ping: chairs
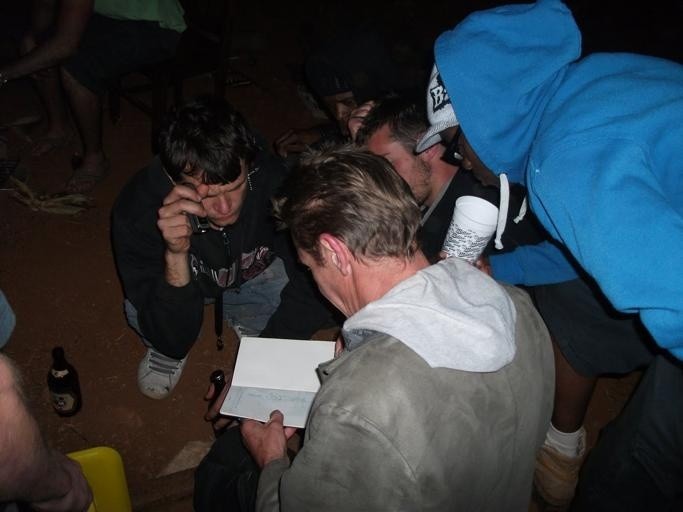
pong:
[106,14,230,157]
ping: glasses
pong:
[440,125,465,167]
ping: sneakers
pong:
[534,430,587,506]
[137,347,191,399]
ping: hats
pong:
[416,63,460,153]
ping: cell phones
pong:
[180,181,210,234]
